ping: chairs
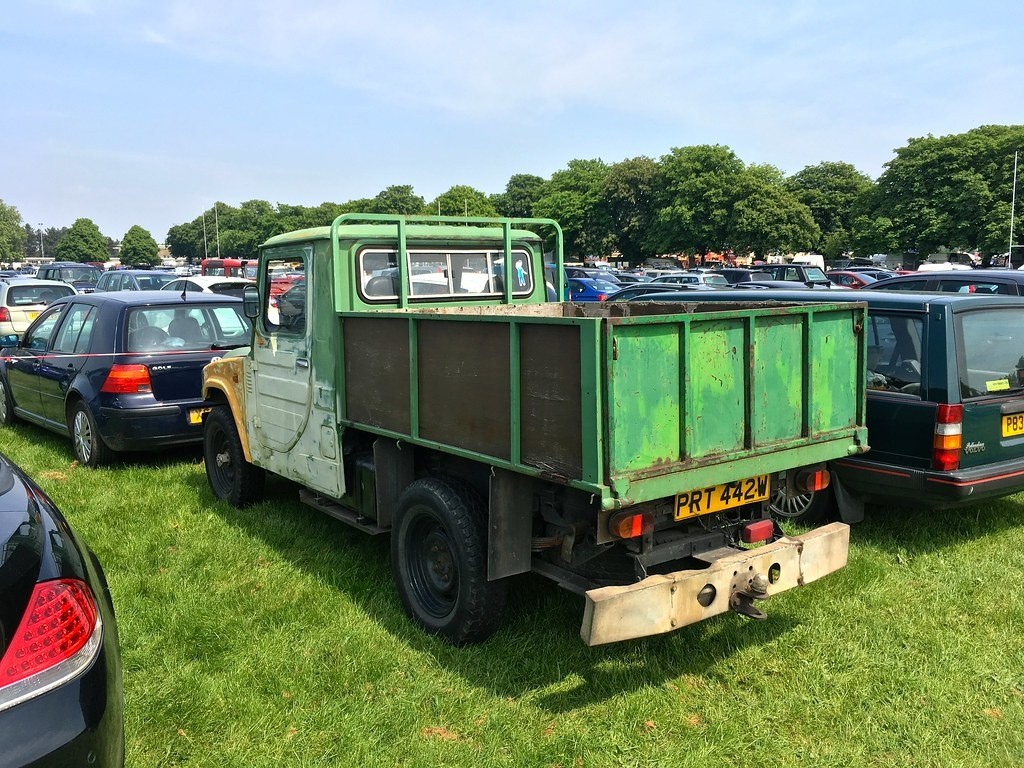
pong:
[366,275,401,298]
[484,274,515,292]
[168,315,204,342]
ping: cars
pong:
[1,260,1024,522]
[0,450,124,768]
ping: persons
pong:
[895,262,903,271]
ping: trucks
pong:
[194,212,872,646]
